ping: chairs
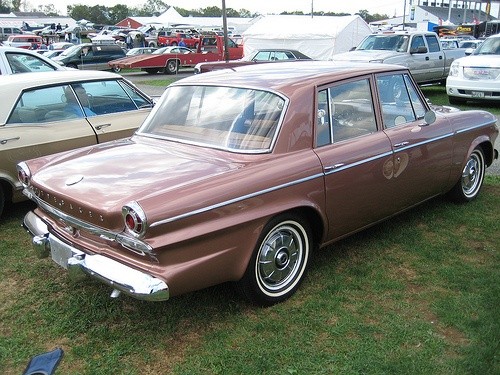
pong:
[18,105,78,123]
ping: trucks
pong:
[108,35,244,75]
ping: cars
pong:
[0,69,163,220]
[126,47,159,57]
[438,28,486,56]
[445,31,500,106]
[16,59,500,307]
[0,23,240,51]
[193,48,313,75]
[0,46,79,76]
[151,46,195,55]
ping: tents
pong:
[243,15,373,61]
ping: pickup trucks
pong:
[329,31,466,85]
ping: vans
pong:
[43,42,127,73]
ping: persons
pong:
[125,34,133,49]
[178,38,186,47]
[57,23,69,30]
[65,85,96,117]
[150,25,156,32]
